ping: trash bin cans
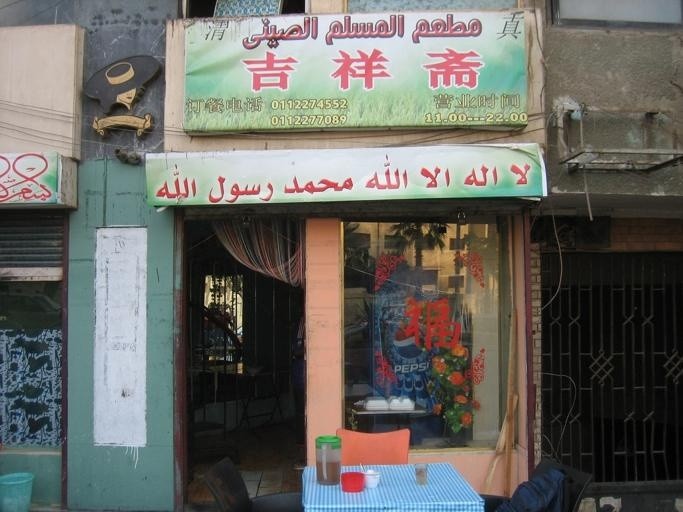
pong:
[0,472,35,512]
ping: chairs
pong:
[479,457,596,510]
[202,454,301,511]
[332,424,411,466]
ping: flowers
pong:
[398,296,481,438]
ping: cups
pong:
[414,463,428,484]
[315,435,380,491]
